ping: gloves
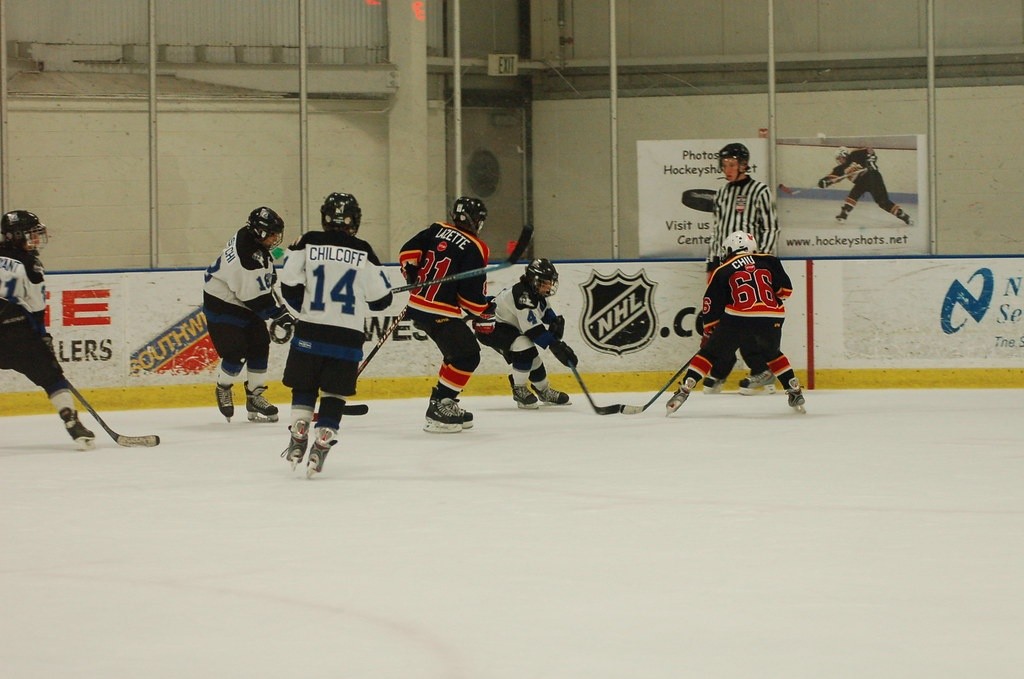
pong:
[273,308,295,329]
[700,332,710,349]
[819,178,832,188]
[868,161,878,172]
[549,341,578,368]
[473,303,497,336]
[548,315,564,340]
[41,333,52,346]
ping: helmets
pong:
[721,230,757,263]
[720,143,749,167]
[321,192,363,230]
[247,207,284,241]
[452,197,488,232]
[0,211,46,255]
[834,146,849,159]
[526,258,558,297]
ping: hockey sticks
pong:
[269,284,369,417]
[391,224,535,294]
[313,304,407,422]
[620,326,715,415]
[57,363,160,447]
[555,336,621,415]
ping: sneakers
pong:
[896,209,912,225]
[281,420,310,470]
[306,427,338,478]
[836,211,848,223]
[423,369,806,433]
[215,382,235,423]
[244,380,279,422]
[59,408,95,449]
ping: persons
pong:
[703,143,782,395]
[818,146,914,225]
[204,206,293,422]
[398,196,497,433]
[664,232,806,417]
[477,258,578,409]
[280,192,393,478]
[0,210,97,450]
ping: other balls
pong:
[660,327,670,337]
